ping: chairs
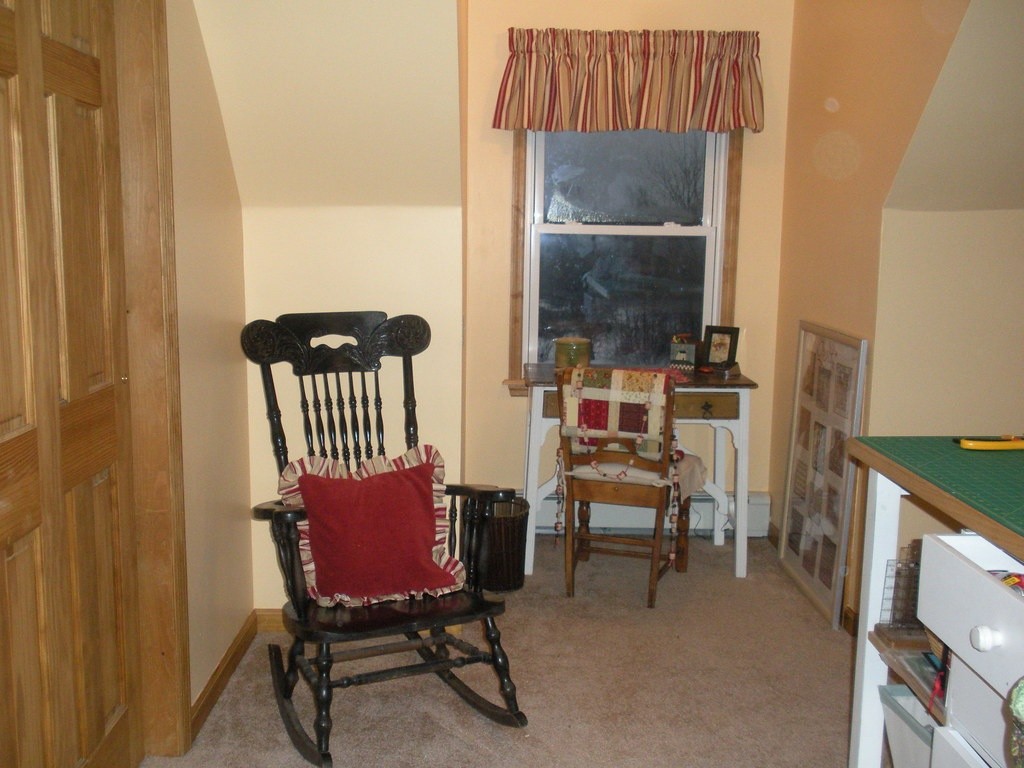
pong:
[553,364,704,609]
[239,309,528,768]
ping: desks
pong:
[843,431,1023,768]
[518,361,758,580]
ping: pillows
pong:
[276,442,466,608]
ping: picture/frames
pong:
[703,325,740,367]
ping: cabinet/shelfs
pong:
[916,526,1022,768]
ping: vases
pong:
[777,319,871,634]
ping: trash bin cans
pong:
[461,496,531,594]
